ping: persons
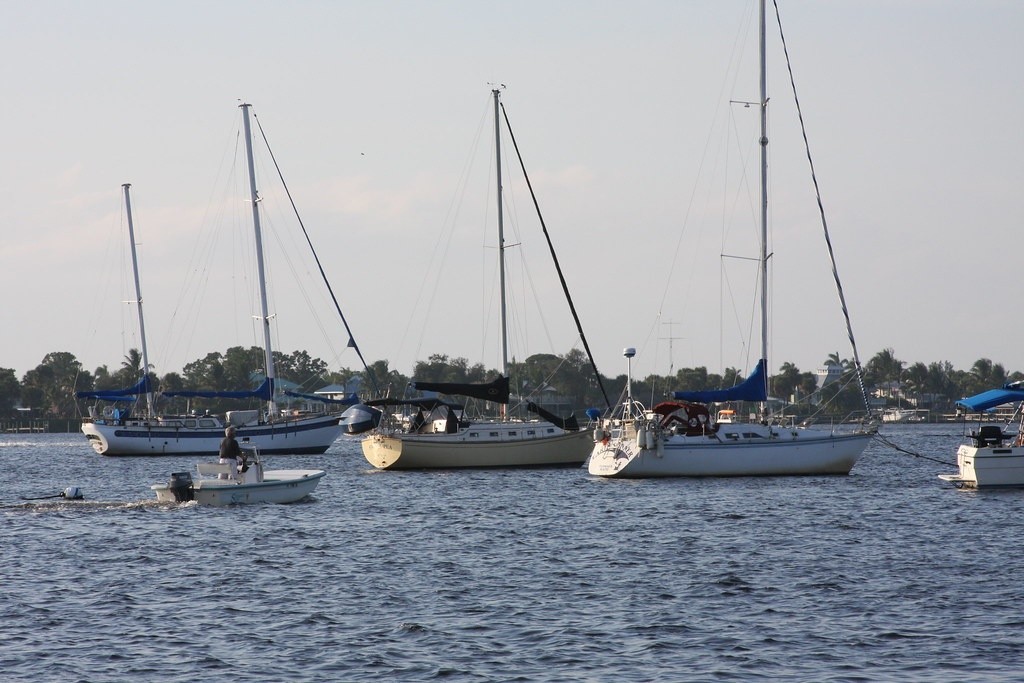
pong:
[191,409,213,418]
[219,426,249,473]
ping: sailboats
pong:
[586,0,885,478]
[80,89,395,458]
[362,79,625,471]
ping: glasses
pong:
[232,432,237,435]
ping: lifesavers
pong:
[103,405,114,418]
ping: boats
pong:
[935,378,1024,490]
[849,403,928,425]
[151,441,326,507]
